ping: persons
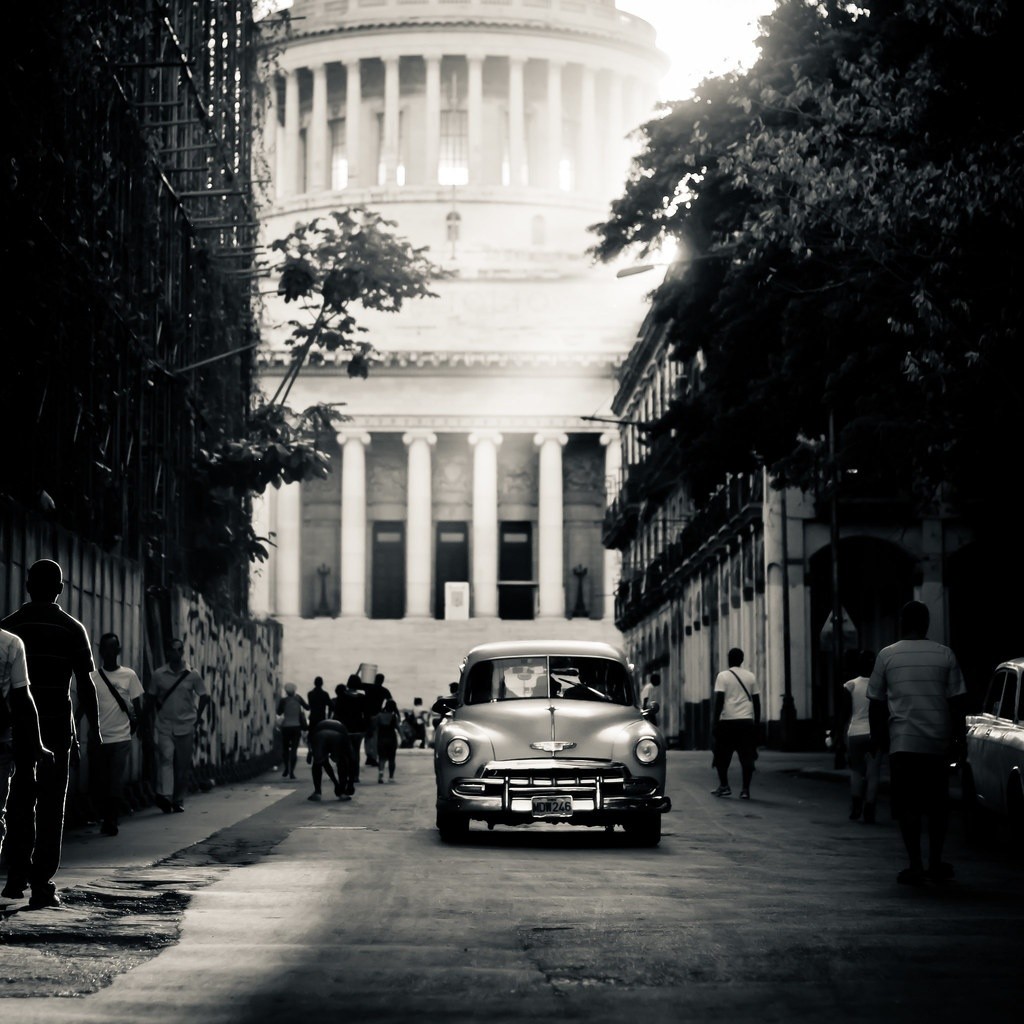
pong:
[866,600,969,888]
[0,559,208,906]
[831,649,881,823]
[710,648,760,798]
[640,674,661,726]
[276,673,458,802]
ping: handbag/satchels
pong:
[130,712,139,735]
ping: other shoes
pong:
[308,792,324,802]
[849,810,876,825]
[154,793,173,814]
[338,795,352,802]
[29,895,59,909]
[895,865,941,885]
[378,770,383,784]
[388,778,395,783]
[711,786,731,797]
[282,769,296,779]
[740,791,750,799]
[100,821,119,836]
[1,885,24,900]
[174,804,185,813]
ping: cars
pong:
[430,636,673,849]
[958,656,1024,849]
